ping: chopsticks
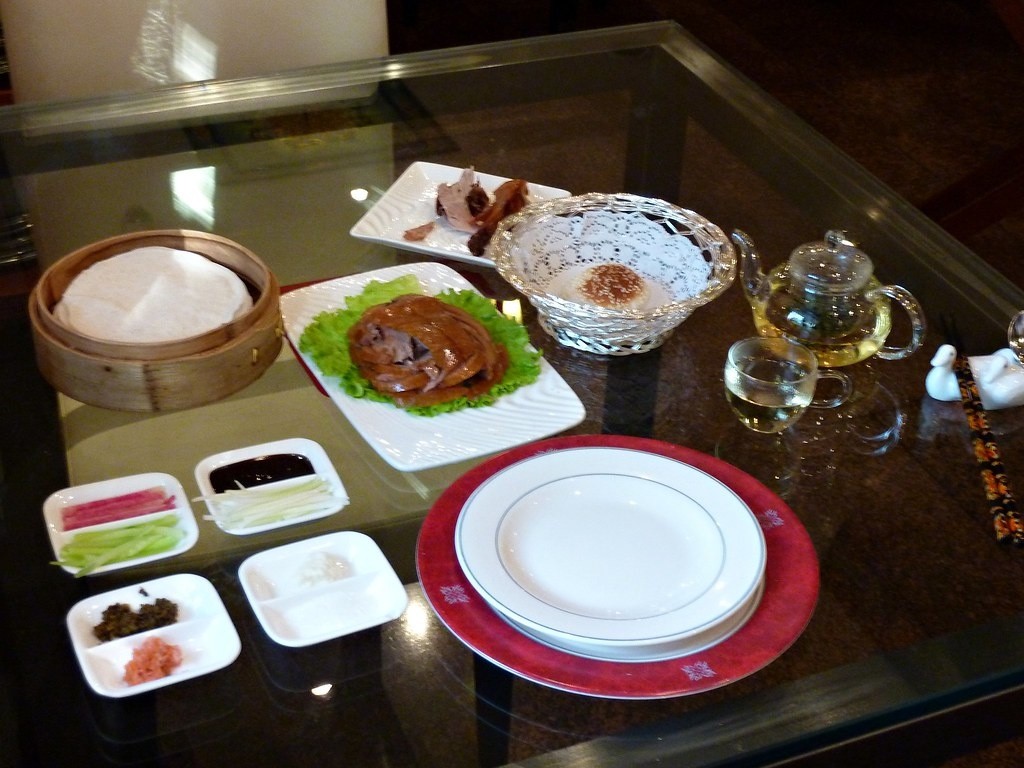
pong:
[938,307,1024,551]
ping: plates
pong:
[349,161,572,268]
[454,446,768,647]
[238,530,409,648]
[195,438,350,536]
[414,433,821,701]
[64,573,242,698]
[278,262,587,473]
[42,472,201,575]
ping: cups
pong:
[724,336,854,434]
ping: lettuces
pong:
[294,277,545,418]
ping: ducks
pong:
[402,165,532,257]
[347,293,510,408]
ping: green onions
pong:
[190,476,352,531]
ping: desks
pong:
[0,20,1024,768]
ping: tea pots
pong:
[732,227,927,370]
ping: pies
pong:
[48,244,253,344]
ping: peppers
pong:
[47,514,189,580]
